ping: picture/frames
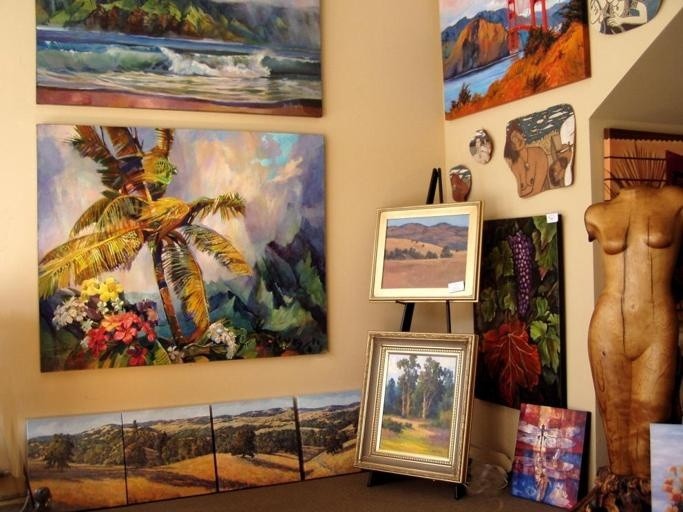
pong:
[366,199,485,303]
[352,329,482,486]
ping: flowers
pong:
[662,464,683,512]
[49,274,175,366]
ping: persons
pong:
[472,136,489,162]
[599,0,648,34]
[504,125,549,197]
[548,156,568,188]
[584,184,683,482]
[588,0,602,27]
